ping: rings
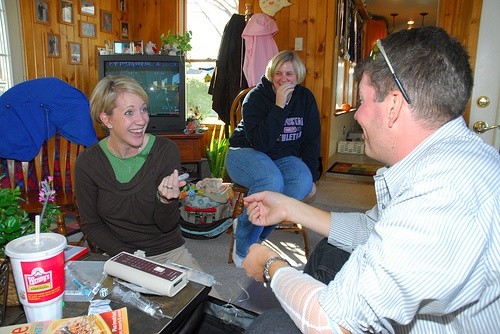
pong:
[167,186,173,190]
[163,185,167,188]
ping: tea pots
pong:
[145,42,157,55]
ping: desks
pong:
[0,253,212,334]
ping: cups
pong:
[5,233,67,323]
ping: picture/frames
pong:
[113,41,144,55]
[100,8,114,34]
[57,0,75,26]
[118,0,128,14]
[32,0,51,26]
[95,45,107,71]
[68,41,83,65]
[78,20,97,39]
[120,20,130,41]
[78,0,96,18]
[44,32,62,59]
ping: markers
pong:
[179,173,189,182]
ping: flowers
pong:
[181,107,207,134]
[0,176,61,259]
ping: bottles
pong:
[194,106,199,117]
[188,109,193,118]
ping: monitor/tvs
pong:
[98,55,186,133]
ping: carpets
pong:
[326,160,384,176]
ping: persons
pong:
[242,27,500,334]
[227,50,324,271]
[74,77,222,300]
[105,62,180,107]
[38,0,142,62]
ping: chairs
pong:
[0,77,99,248]
[226,85,316,264]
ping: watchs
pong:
[263,257,291,284]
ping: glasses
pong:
[370,39,411,104]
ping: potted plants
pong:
[160,28,193,56]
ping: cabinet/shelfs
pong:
[364,20,388,59]
[97,133,202,183]
